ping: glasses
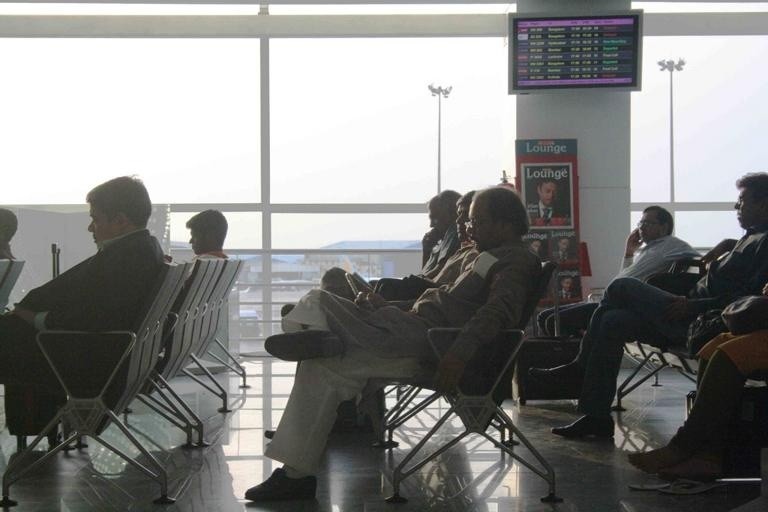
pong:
[464,216,497,227]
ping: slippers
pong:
[658,478,715,495]
[628,476,671,490]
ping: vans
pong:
[239,310,263,337]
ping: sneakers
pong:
[264,329,342,361]
[245,467,318,504]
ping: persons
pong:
[528,178,562,218]
[536,204,694,340]
[243,185,546,502]
[555,236,574,264]
[559,277,575,298]
[527,172,768,439]
[169,208,231,320]
[0,208,19,259]
[0,175,166,400]
[626,281,768,482]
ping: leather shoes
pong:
[528,361,582,386]
[551,414,615,439]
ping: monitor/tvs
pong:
[507,8,644,93]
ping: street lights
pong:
[658,57,687,235]
[428,81,454,194]
